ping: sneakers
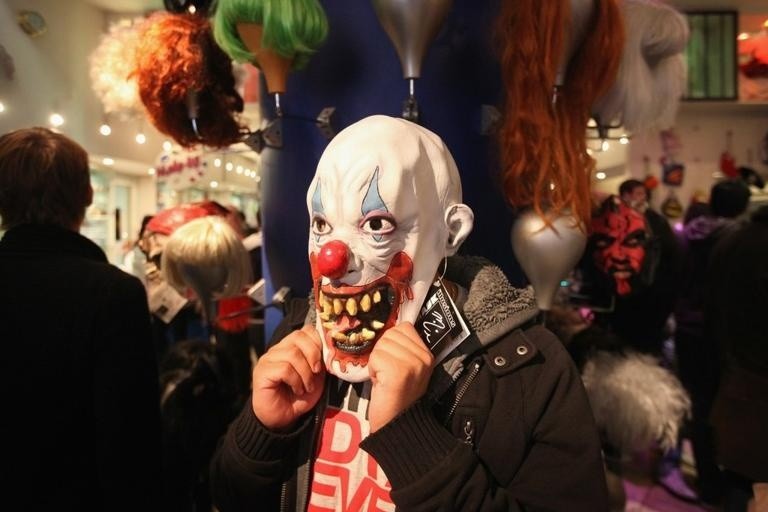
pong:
[656,463,698,503]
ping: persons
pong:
[210,115,609,512]
[552,166,768,512]
[0,126,263,511]
